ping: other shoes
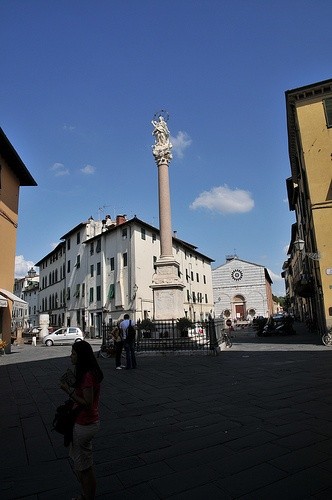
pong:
[114,367,122,370]
[121,365,126,367]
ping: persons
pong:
[150,114,170,144]
[110,314,138,370]
[57,341,104,500]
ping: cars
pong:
[276,314,283,318]
[43,326,85,347]
[22,326,41,339]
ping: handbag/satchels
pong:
[53,393,75,436]
[125,320,136,345]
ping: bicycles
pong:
[221,328,232,348]
[99,338,127,359]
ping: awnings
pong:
[0,288,28,310]
